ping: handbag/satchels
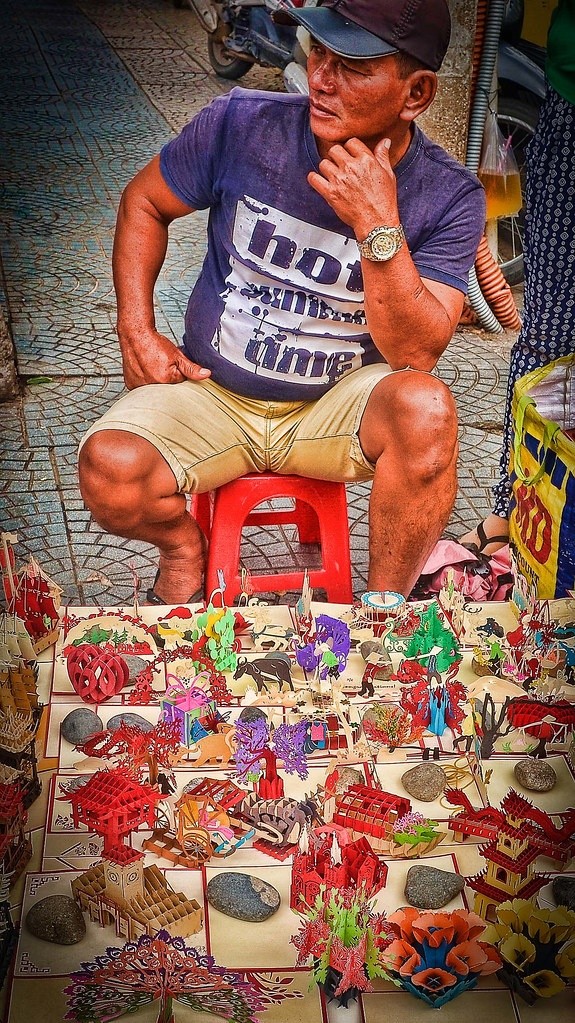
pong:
[509,352,575,599]
[473,112,524,220]
[413,535,515,601]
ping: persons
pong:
[77,1,487,605]
[459,0,575,562]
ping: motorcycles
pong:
[187,0,548,288]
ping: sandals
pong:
[450,522,509,563]
[147,570,204,605]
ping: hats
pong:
[269,1,451,72]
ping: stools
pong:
[191,473,357,608]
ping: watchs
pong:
[357,224,405,262]
